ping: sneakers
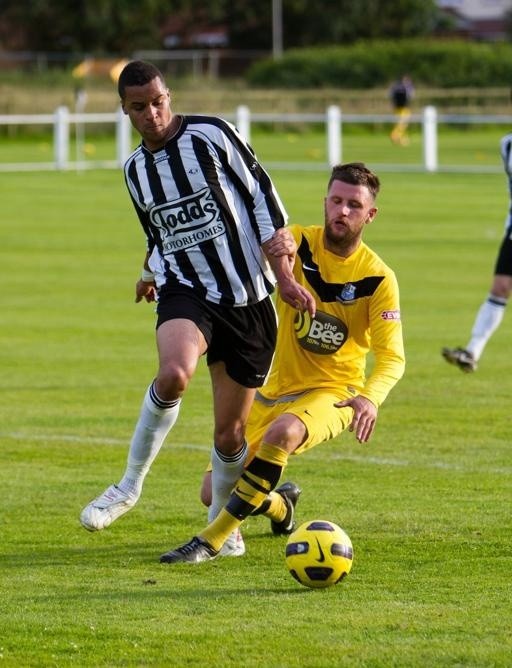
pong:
[77,481,146,535]
[270,480,303,535]
[441,345,479,375]
[213,534,248,560]
[158,536,221,567]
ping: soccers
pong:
[285,520,353,589]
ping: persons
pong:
[439,129,512,373]
[161,160,408,562]
[77,58,317,555]
[386,71,415,144]
